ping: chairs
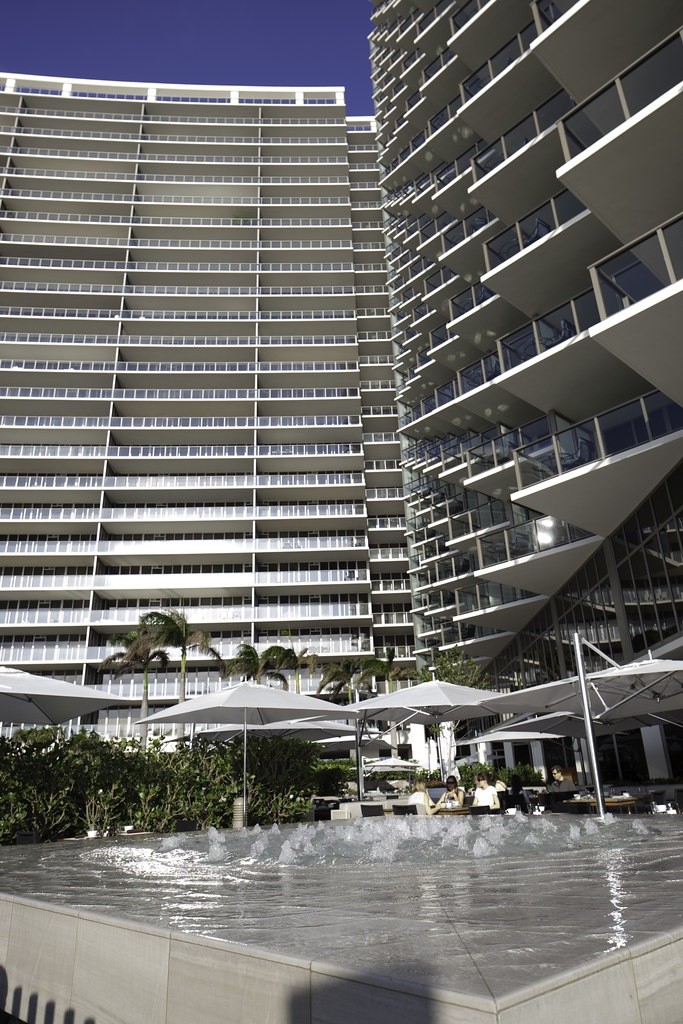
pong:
[468,805,491,814]
[361,805,385,816]
[393,806,417,815]
[497,784,683,815]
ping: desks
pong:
[116,832,154,836]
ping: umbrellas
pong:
[0,663,135,728]
[134,657,682,824]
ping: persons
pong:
[425,788,436,808]
[508,773,534,814]
[549,765,578,792]
[436,776,465,810]
[490,773,507,792]
[471,771,500,810]
[408,780,442,816]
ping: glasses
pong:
[447,781,454,784]
[552,772,559,775]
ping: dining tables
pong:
[563,797,638,815]
[431,806,469,815]
